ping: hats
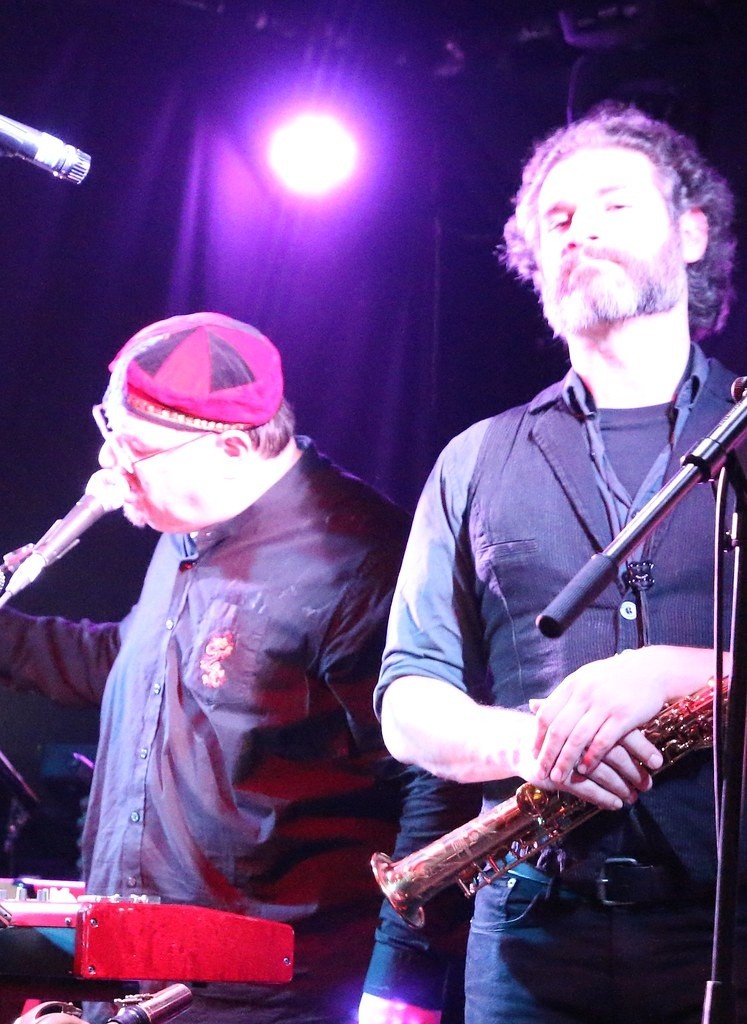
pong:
[108,312,284,434]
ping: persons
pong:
[0,309,486,1024]
[374,98,747,1024]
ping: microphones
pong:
[4,470,130,598]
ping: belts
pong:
[501,848,747,905]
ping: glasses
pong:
[92,403,212,474]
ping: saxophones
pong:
[372,673,735,929]
